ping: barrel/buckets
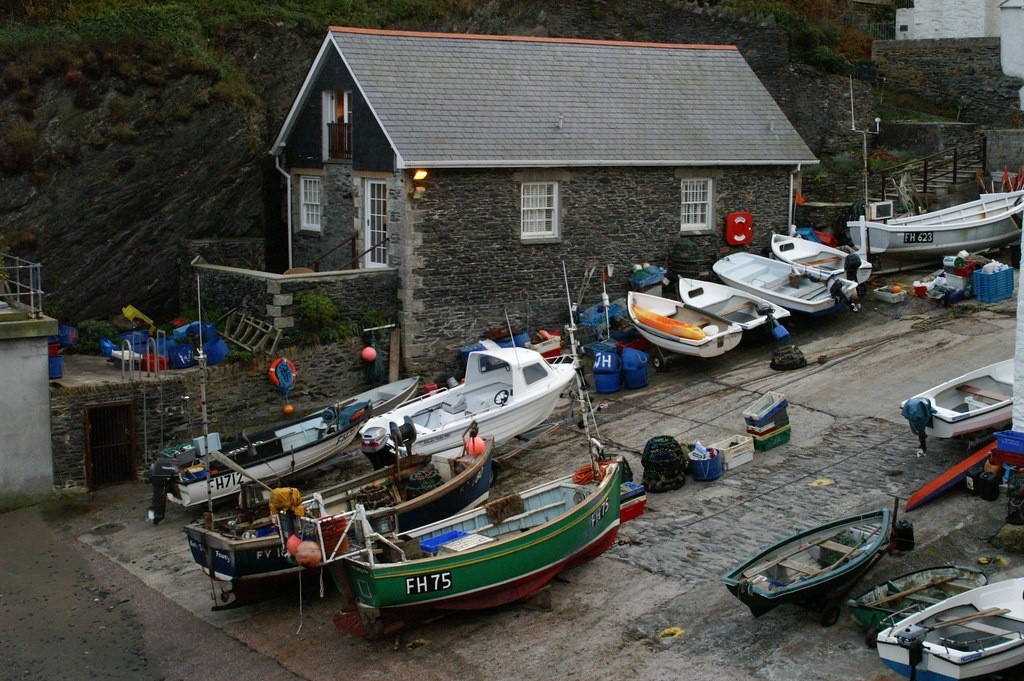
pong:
[690,449,723,481]
[461,329,531,374]
[182,466,208,481]
[593,348,650,393]
[48,326,77,379]
[101,321,230,371]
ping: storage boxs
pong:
[100,304,231,370]
[484,324,516,340]
[795,227,838,246]
[160,444,197,466]
[990,429,1024,467]
[709,390,792,470]
[915,260,1015,307]
[873,285,907,303]
[620,481,647,523]
[377,529,495,564]
[524,335,561,359]
[298,515,348,557]
[48,325,78,379]
[964,395,999,411]
[581,266,666,358]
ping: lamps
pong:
[408,187,425,200]
[412,169,427,180]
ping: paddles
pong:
[211,451,272,491]
[815,526,882,576]
[867,576,958,607]
[743,529,847,578]
[924,607,1012,631]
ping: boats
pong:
[144,307,579,612]
[770,233,872,286]
[627,290,744,359]
[332,259,622,631]
[898,358,1016,455]
[846,76,1024,262]
[712,251,861,318]
[719,507,891,619]
[677,274,791,345]
[875,577,1024,681]
[846,563,987,647]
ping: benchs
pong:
[885,580,942,604]
[800,257,842,266]
[808,538,862,555]
[790,281,825,298]
[956,385,1011,401]
[694,320,709,326]
[767,556,820,574]
[937,615,1023,639]
[714,301,755,318]
[760,275,789,290]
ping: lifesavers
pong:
[269,358,297,386]
